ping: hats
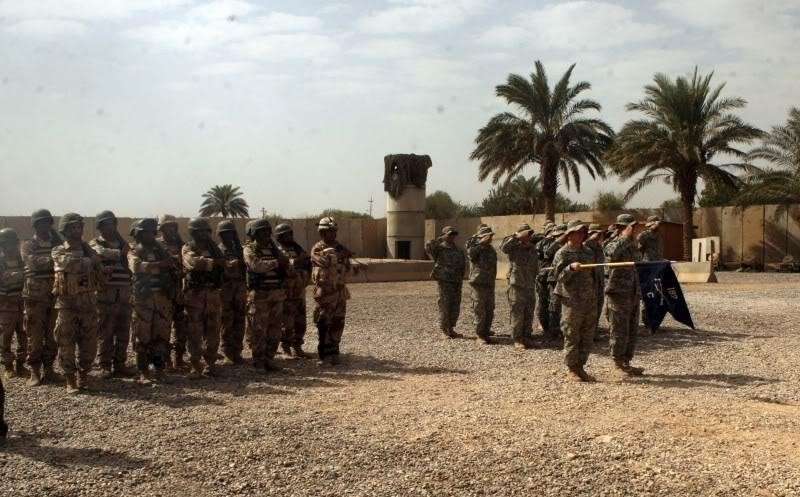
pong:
[645,216,662,226]
[614,214,639,226]
[471,224,495,238]
[519,220,606,237]
[442,226,459,236]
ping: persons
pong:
[552,219,600,383]
[1,208,311,395]
[311,216,352,365]
[601,213,644,378]
[424,225,465,340]
[465,212,663,351]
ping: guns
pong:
[235,229,243,268]
[292,240,311,268]
[83,239,97,257]
[333,238,365,270]
[129,228,182,277]
[115,230,128,252]
[50,228,63,244]
[270,238,289,267]
[208,237,223,258]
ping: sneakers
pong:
[5,359,138,395]
[613,359,645,378]
[441,329,544,349]
[320,349,343,364]
[253,342,307,372]
[569,366,589,382]
[141,353,247,385]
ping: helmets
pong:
[188,217,212,232]
[30,209,54,225]
[217,220,234,232]
[58,213,85,233]
[95,211,117,230]
[0,228,18,243]
[275,223,293,235]
[129,215,179,240]
[318,216,338,231]
[245,220,272,238]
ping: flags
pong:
[633,260,695,335]
